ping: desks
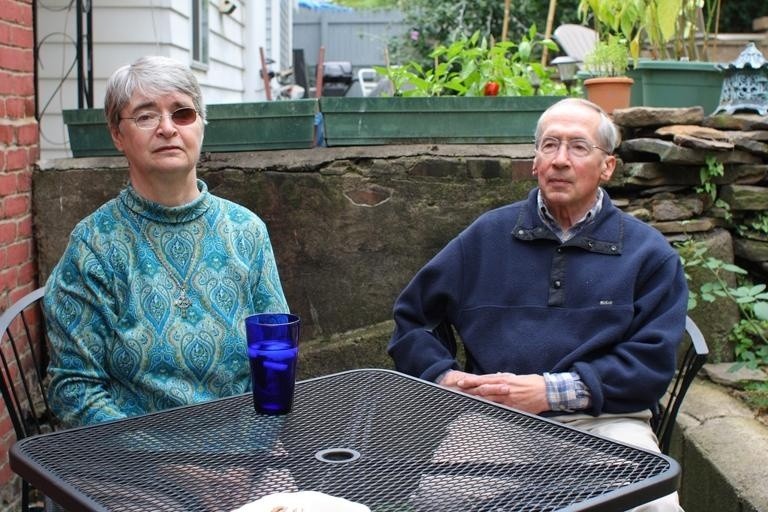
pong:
[9,368,682,512]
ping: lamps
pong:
[549,56,583,94]
[527,66,541,95]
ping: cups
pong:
[244,313,301,415]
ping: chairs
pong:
[422,316,710,456]
[0,286,63,512]
[359,68,389,97]
[552,21,624,74]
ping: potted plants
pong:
[577,0,725,117]
[320,21,584,147]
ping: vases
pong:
[63,99,318,157]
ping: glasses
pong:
[537,137,611,158]
[118,107,200,129]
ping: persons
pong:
[384,91,693,512]
[42,51,301,512]
